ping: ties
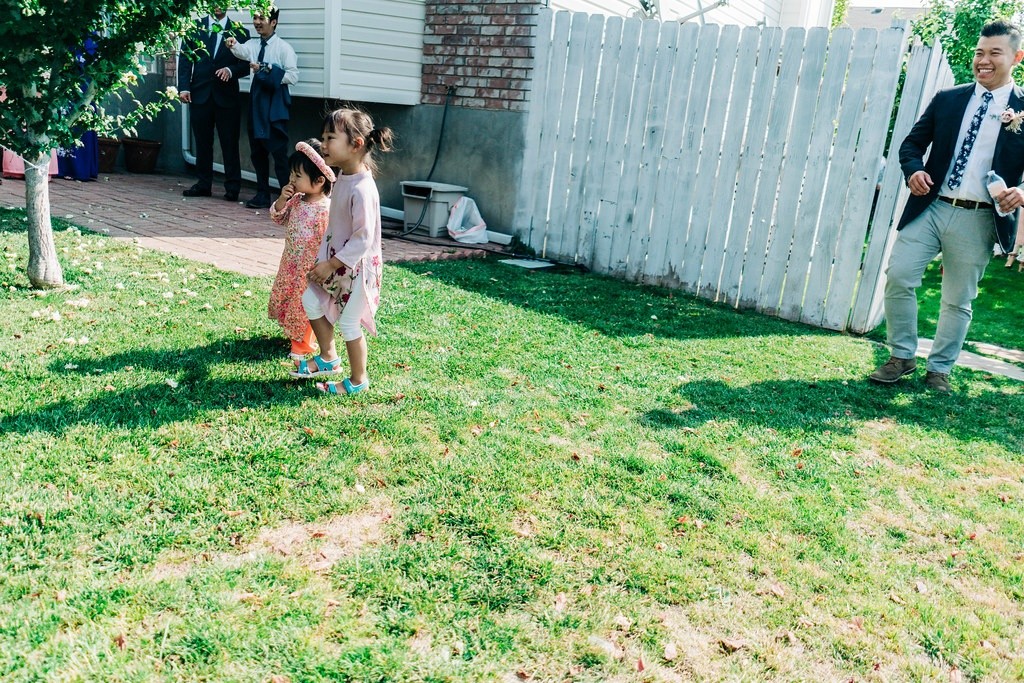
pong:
[258,42,267,63]
[948,92,992,191]
[209,23,216,59]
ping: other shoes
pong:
[224,192,238,201]
[183,180,212,196]
[246,193,270,208]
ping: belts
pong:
[936,195,993,209]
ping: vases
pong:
[98,136,121,174]
[122,137,163,175]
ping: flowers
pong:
[999,105,1024,134]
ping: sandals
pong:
[316,377,368,395]
[288,356,342,378]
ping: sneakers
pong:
[869,357,916,382]
[925,371,951,392]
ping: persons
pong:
[288,106,394,397]
[177,1,252,201]
[267,138,338,361]
[868,21,1024,392]
[225,2,299,209]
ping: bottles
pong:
[986,170,1015,217]
